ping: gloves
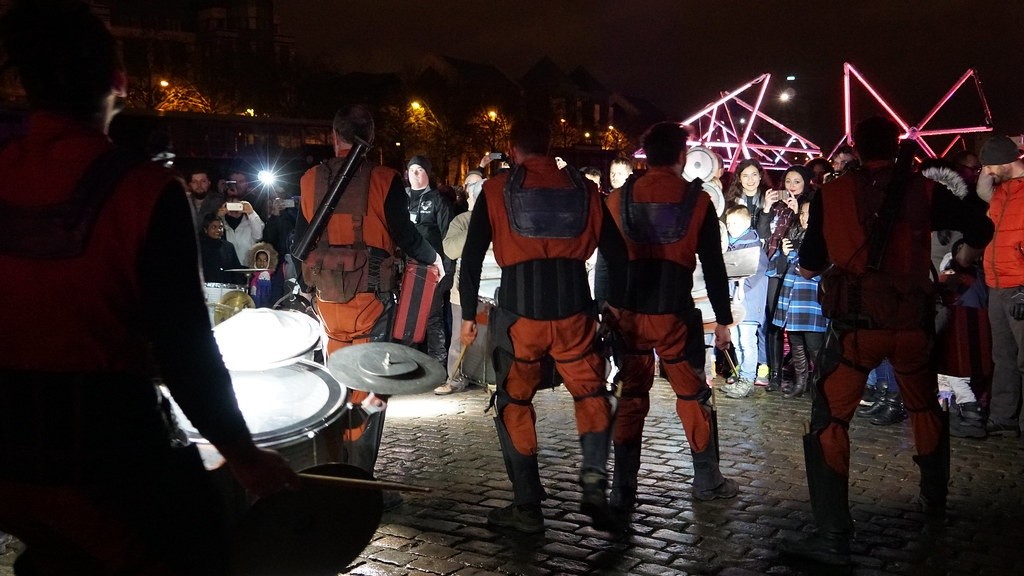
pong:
[1009,285,1024,321]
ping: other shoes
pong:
[486,500,544,534]
[947,415,987,439]
[433,381,463,394]
[983,418,1021,439]
[778,535,851,564]
[720,381,739,392]
[579,492,615,531]
[755,365,769,384]
[692,476,738,500]
[610,489,636,513]
[728,365,740,383]
[917,490,946,515]
[726,377,754,398]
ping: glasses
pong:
[963,164,979,174]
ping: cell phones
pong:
[787,239,801,249]
[227,203,243,211]
[489,153,502,159]
[771,190,789,200]
[226,181,236,192]
[280,200,295,207]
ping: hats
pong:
[979,135,1020,167]
[407,155,431,180]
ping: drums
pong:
[203,280,248,327]
[458,278,564,390]
[157,307,348,479]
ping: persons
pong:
[595,122,739,517]
[242,251,284,309]
[0,0,1024,576]
[798,120,997,550]
[459,119,630,540]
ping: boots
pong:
[778,336,795,393]
[763,333,784,391]
[783,344,811,398]
[870,390,904,425]
[857,383,889,418]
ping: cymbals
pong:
[247,461,383,576]
[214,290,256,328]
[327,339,448,395]
